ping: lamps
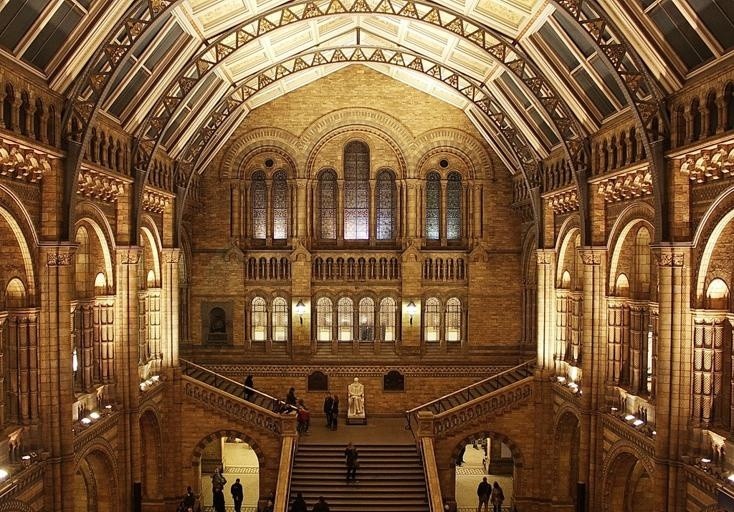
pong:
[406,301,417,325]
[295,299,306,324]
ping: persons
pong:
[348,377,364,416]
[298,399,311,433]
[231,479,243,512]
[477,476,492,512]
[243,374,254,401]
[324,392,334,429]
[286,387,297,406]
[312,496,329,512]
[491,481,504,512]
[331,395,339,431]
[344,441,360,483]
[212,467,227,505]
[213,487,226,512]
[291,492,307,512]
